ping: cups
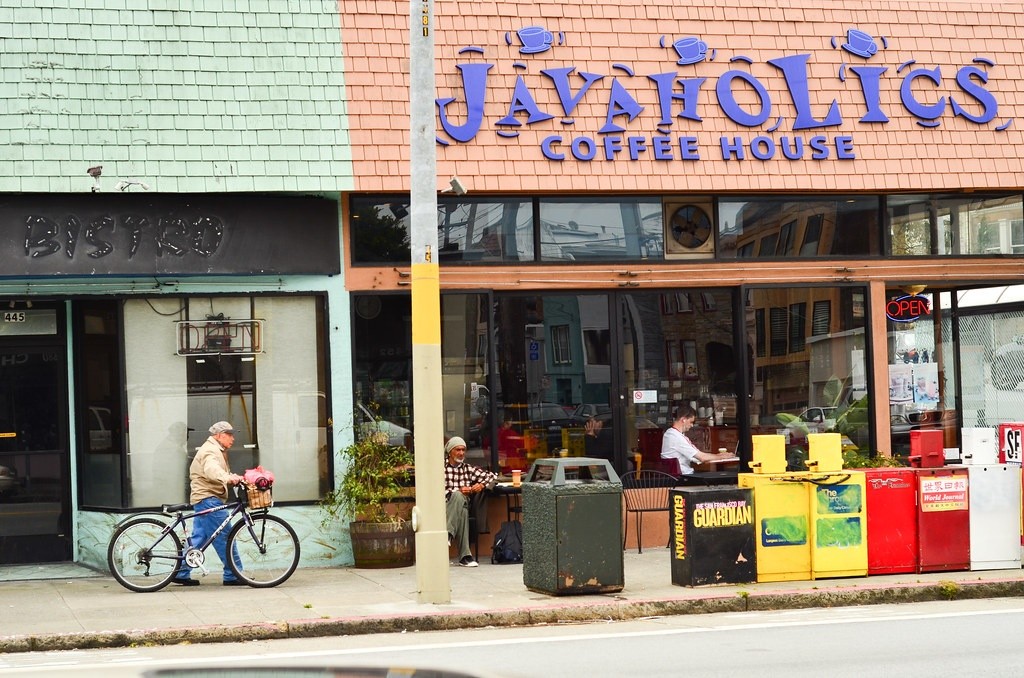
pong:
[512,470,521,487]
[718,448,728,453]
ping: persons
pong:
[444,437,495,567]
[584,415,613,480]
[660,405,735,486]
[483,412,539,474]
[171,421,255,586]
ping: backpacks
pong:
[490,520,523,565]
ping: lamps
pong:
[115,180,150,191]
[88,164,103,192]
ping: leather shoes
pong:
[223,578,248,586]
[171,576,200,586]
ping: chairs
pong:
[654,458,681,478]
[620,470,678,554]
[469,484,478,562]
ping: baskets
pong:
[244,482,274,510]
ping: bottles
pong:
[552,447,568,458]
[699,385,728,426]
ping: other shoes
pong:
[458,555,479,567]
[448,532,453,547]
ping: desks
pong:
[680,457,743,486]
[496,484,523,521]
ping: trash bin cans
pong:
[520,456,625,596]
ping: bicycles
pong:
[108,479,300,593]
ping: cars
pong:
[464,384,490,447]
[991,342,1024,390]
[480,403,661,465]
[783,406,921,456]
[88,407,113,451]
[318,391,413,478]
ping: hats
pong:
[445,436,467,453]
[208,421,236,434]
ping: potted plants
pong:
[319,391,415,569]
